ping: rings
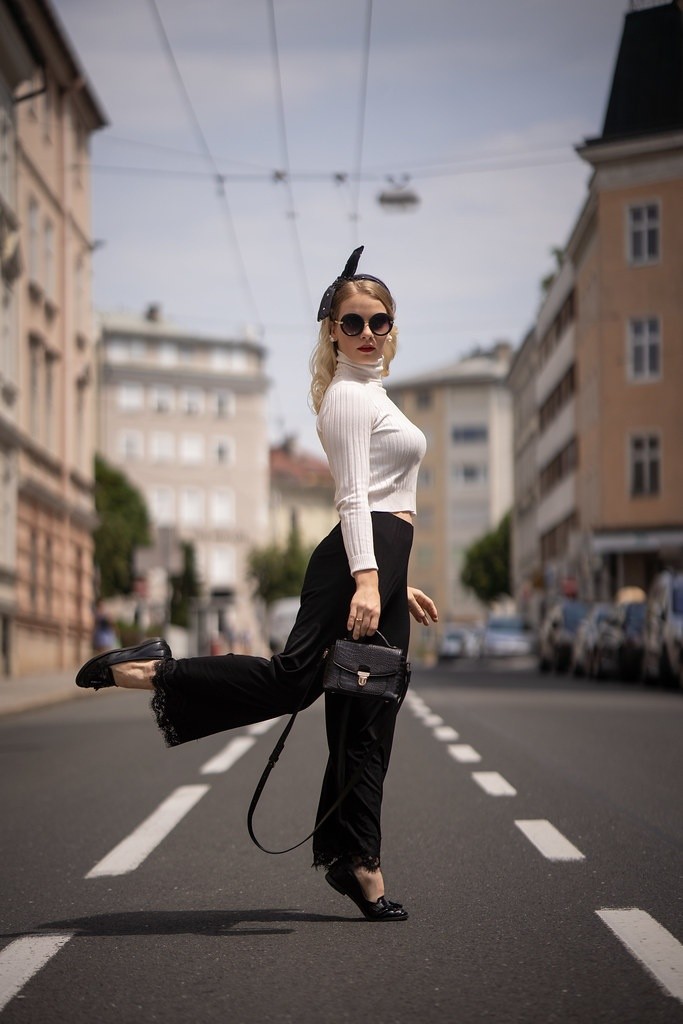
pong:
[356,618,362,621]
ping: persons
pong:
[557,580,589,670]
[74,246,439,921]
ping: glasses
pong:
[330,312,394,336]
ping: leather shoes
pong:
[75,638,171,690]
[325,858,409,921]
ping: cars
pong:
[539,565,683,686]
[441,617,536,662]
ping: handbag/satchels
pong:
[323,628,411,708]
